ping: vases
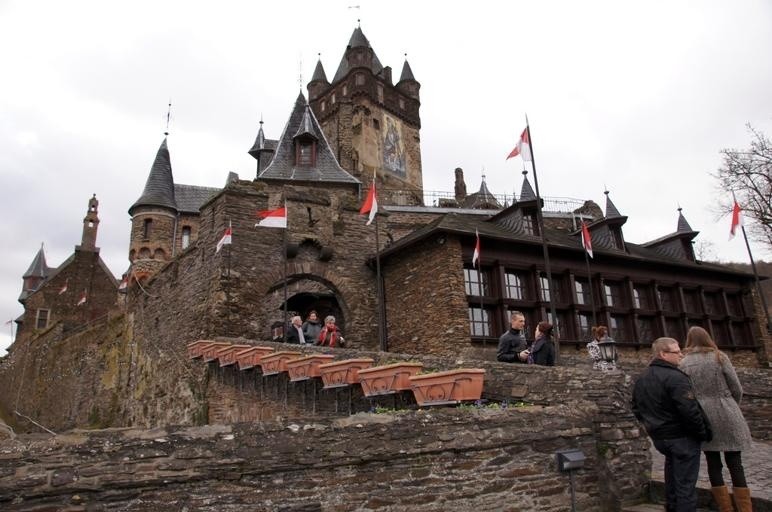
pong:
[185,340,375,390]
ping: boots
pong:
[712,486,734,512]
[732,486,753,512]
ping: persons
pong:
[680,326,755,512]
[313,315,345,348]
[303,310,321,346]
[497,312,533,364]
[285,316,306,346]
[631,337,709,512]
[527,322,558,368]
[585,325,618,370]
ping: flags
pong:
[254,206,286,228]
[359,177,378,226]
[5,321,13,325]
[506,127,533,161]
[59,284,67,296]
[76,291,86,306]
[118,278,129,290]
[216,225,230,253]
[579,218,594,259]
[471,228,480,269]
[728,202,743,242]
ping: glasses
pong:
[664,349,681,355]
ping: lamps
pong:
[557,449,585,471]
[595,330,617,360]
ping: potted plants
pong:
[408,368,486,407]
[356,358,423,399]
